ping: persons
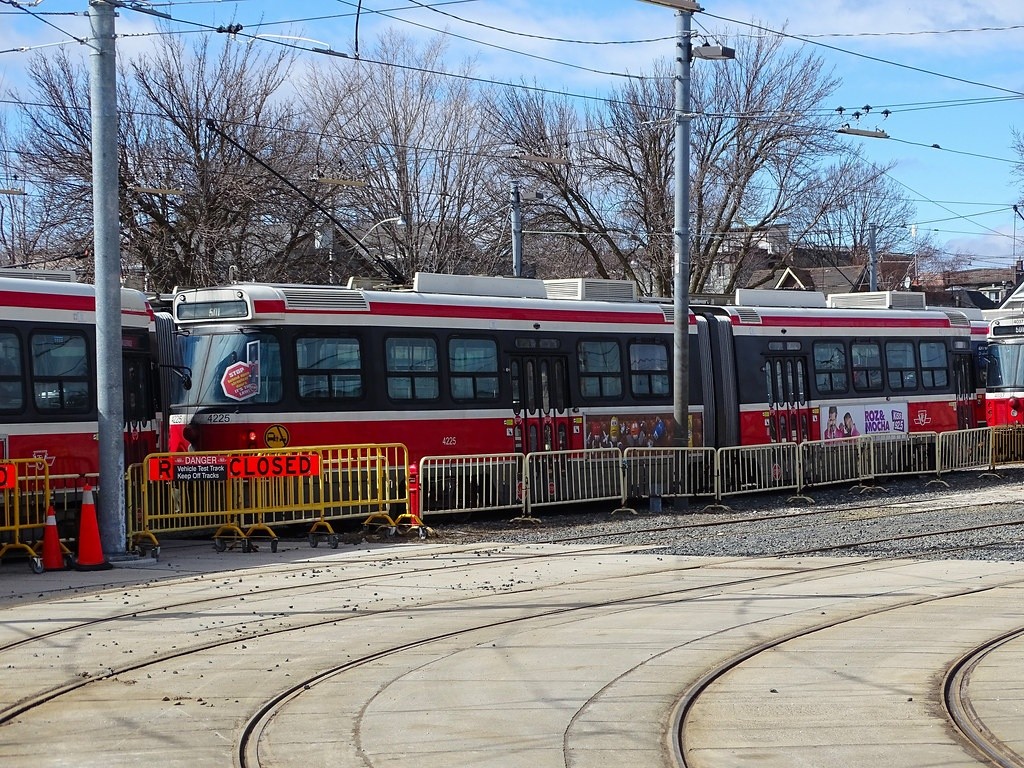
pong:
[839,412,860,443]
[824,406,843,445]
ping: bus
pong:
[0,269,1024,557]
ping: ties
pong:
[833,432,834,439]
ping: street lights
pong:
[673,43,735,514]
[330,182,406,285]
[511,181,544,279]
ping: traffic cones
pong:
[72,485,114,572]
[38,504,69,572]
